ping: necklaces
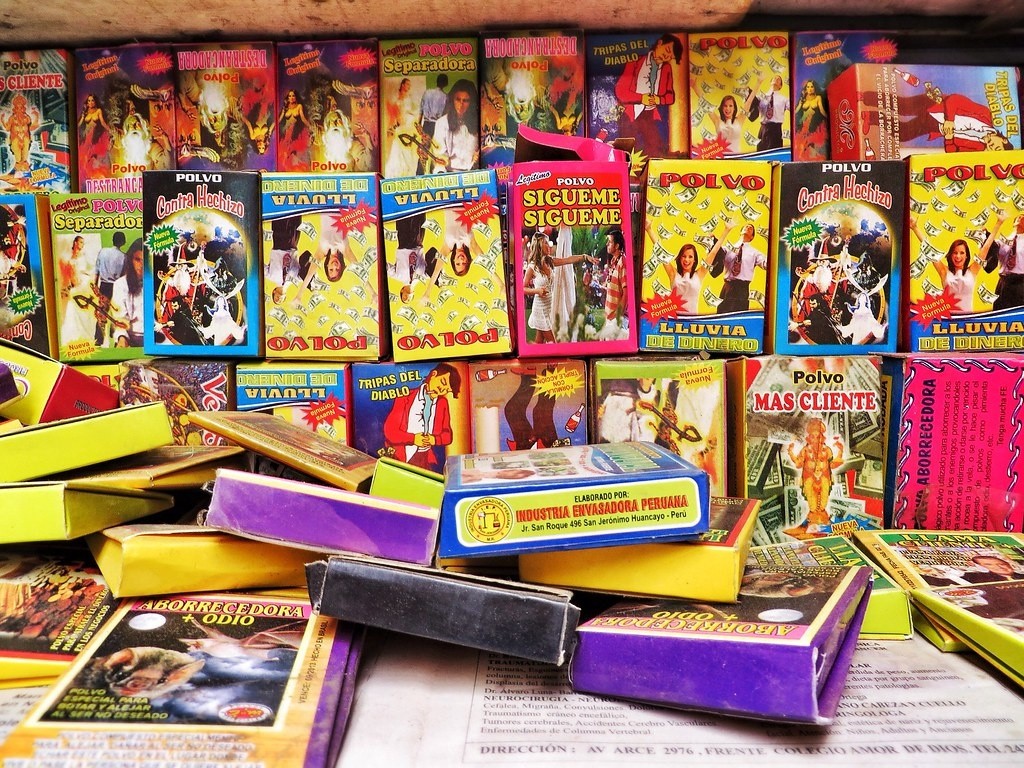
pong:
[606,252,623,283]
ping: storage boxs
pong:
[0,29,1022,727]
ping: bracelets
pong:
[583,254,587,261]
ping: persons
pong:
[483,56,583,137]
[79,95,172,170]
[523,229,627,345]
[264,213,378,309]
[59,232,144,347]
[909,211,1024,314]
[178,69,275,155]
[972,555,1024,582]
[280,90,375,172]
[690,73,826,162]
[385,207,506,305]
[383,74,479,180]
[646,218,767,314]
